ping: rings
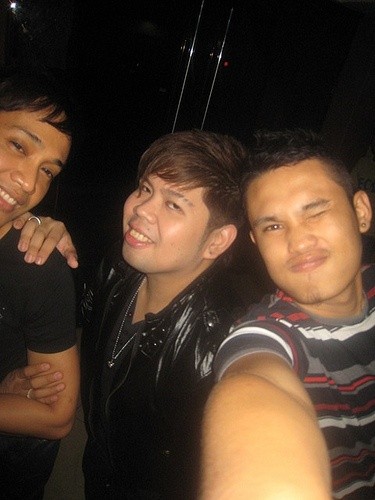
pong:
[27,388,33,400]
[28,216,42,225]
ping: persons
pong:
[201,129,375,500]
[13,130,250,500]
[0,69,81,500]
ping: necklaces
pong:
[108,275,148,367]
